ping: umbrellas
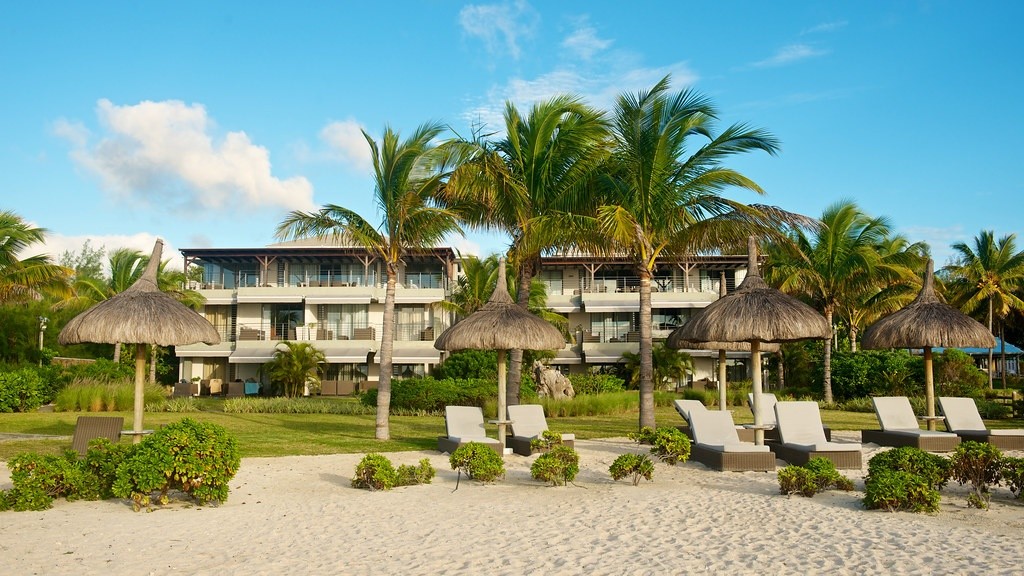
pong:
[676,236,833,445]
[434,258,566,448]
[860,259,997,430]
[57,238,222,444]
[665,326,780,411]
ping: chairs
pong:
[862,396,960,452]
[938,396,1024,451]
[686,410,775,472]
[506,405,575,456]
[69,416,124,456]
[437,405,503,460]
[764,401,862,468]
[748,393,831,444]
[674,400,707,426]
